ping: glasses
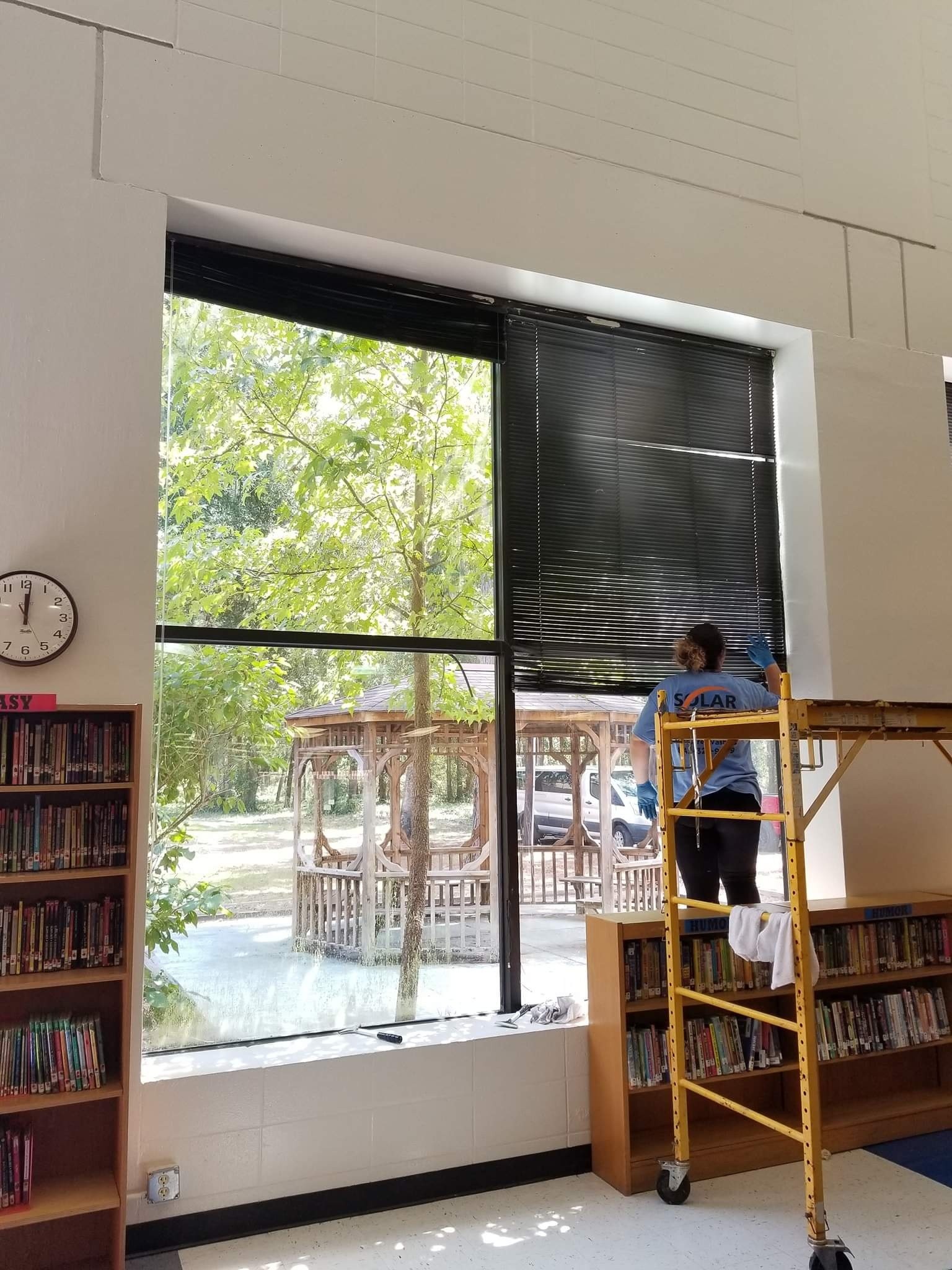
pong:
[723,638,728,644]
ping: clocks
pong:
[0,569,78,666]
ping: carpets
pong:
[862,1130,952,1190]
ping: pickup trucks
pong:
[757,793,786,854]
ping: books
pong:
[810,917,952,980]
[625,938,668,1002]
[626,1020,672,1089]
[0,713,130,786]
[683,1010,784,1081]
[0,892,125,978]
[814,986,950,1062]
[679,936,773,994]
[0,795,128,874]
[0,1121,35,1209]
[0,1008,108,1096]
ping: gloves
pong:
[636,781,659,822]
[745,633,775,670]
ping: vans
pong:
[516,765,659,849]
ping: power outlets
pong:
[146,1166,180,1205]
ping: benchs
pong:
[303,879,490,919]
[563,874,658,911]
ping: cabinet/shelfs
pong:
[0,703,143,1270]
[586,891,952,1196]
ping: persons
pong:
[628,623,782,910]
